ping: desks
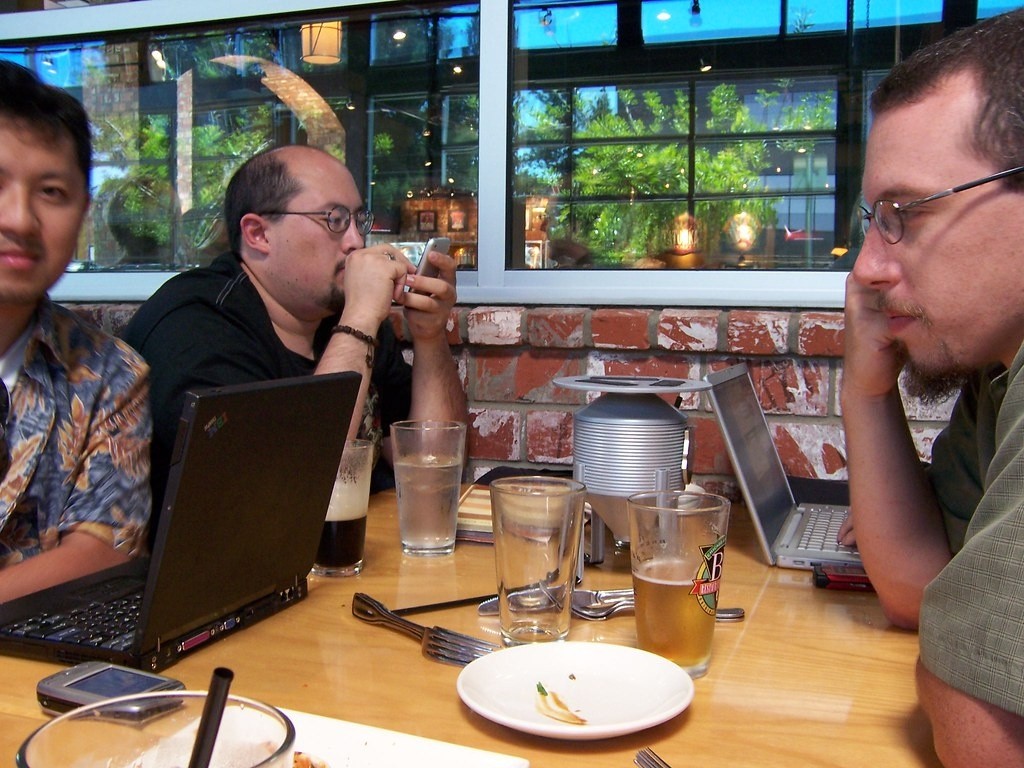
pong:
[0,485,942,768]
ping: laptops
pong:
[0,369,362,675]
[702,362,864,571]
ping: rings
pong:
[385,251,397,262]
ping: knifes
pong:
[476,583,635,616]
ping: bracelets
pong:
[332,325,379,369]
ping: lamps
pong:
[42,1,831,250]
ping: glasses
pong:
[855,164,1023,243]
[258,205,375,237]
[0,375,10,483]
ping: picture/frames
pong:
[544,240,556,269]
[418,210,438,232]
[448,210,469,232]
[525,240,543,268]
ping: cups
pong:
[390,418,466,559]
[628,490,731,679]
[310,439,374,577]
[490,476,587,647]
[16,690,295,768]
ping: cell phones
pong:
[809,566,877,595]
[409,237,450,296]
[35,660,187,724]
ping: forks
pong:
[352,592,501,666]
[633,746,672,768]
[537,579,746,623]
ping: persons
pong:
[0,56,153,606]
[119,143,470,548]
[840,3,1024,767]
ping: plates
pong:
[456,641,694,740]
